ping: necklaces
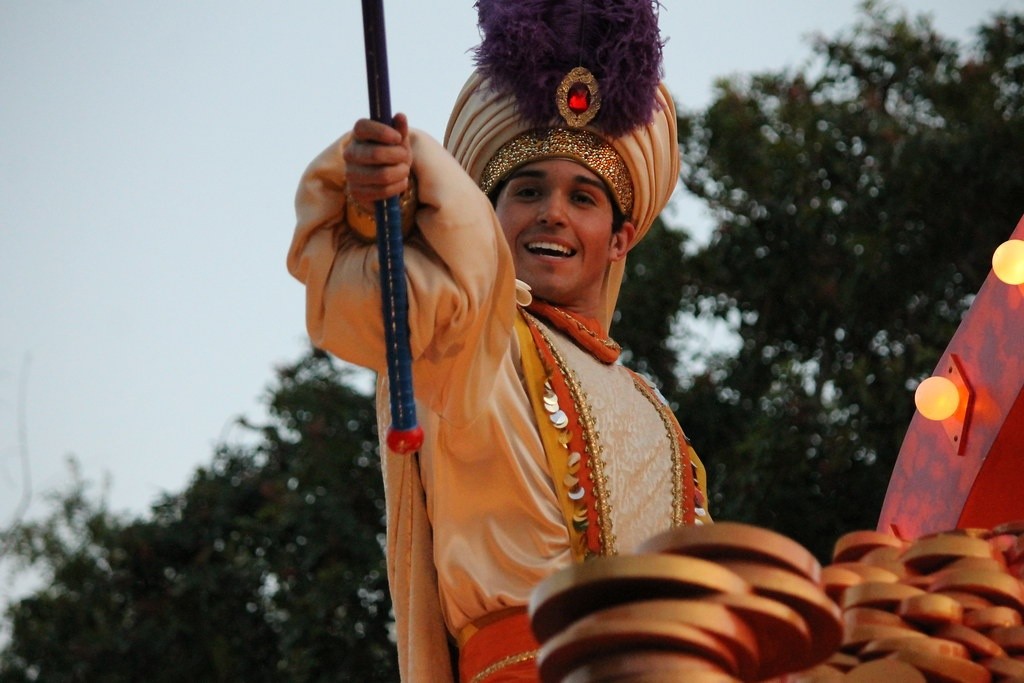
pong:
[555,307,623,351]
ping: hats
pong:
[442,0,681,253]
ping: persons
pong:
[285,0,715,683]
